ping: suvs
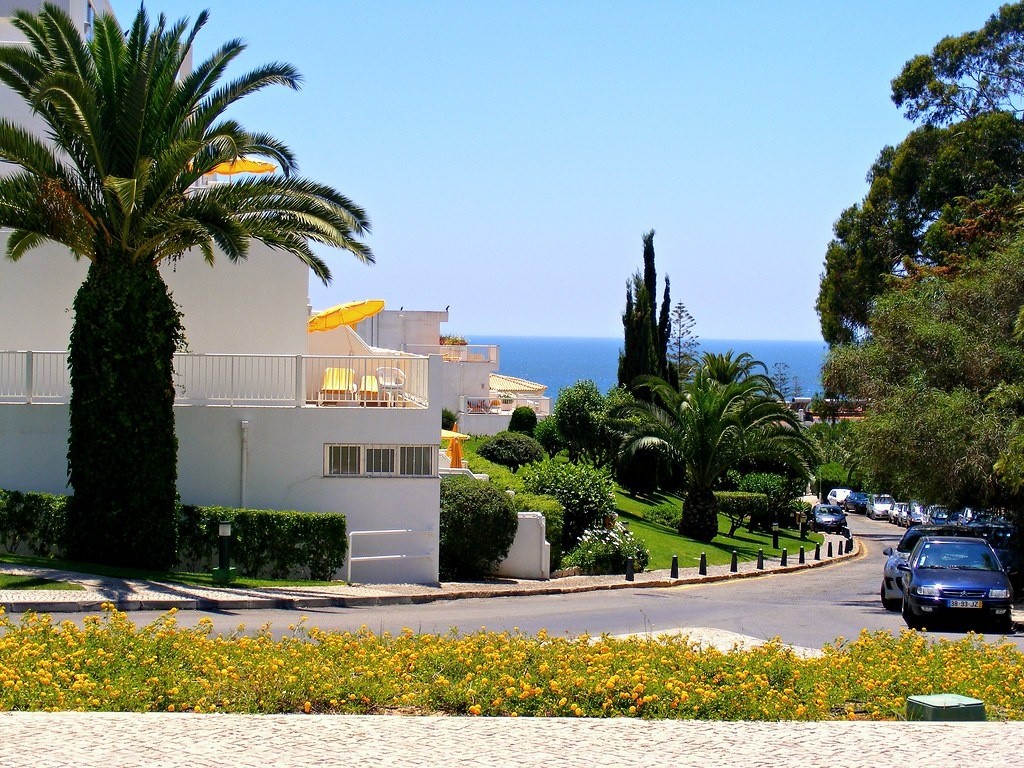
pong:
[880,523,983,612]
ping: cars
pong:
[843,491,869,515]
[826,488,854,510]
[886,501,1024,583]
[865,493,897,522]
[896,535,1016,632]
[809,502,849,535]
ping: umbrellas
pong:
[307,299,385,356]
[445,422,464,468]
[186,158,278,188]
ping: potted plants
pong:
[500,395,514,410]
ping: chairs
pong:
[318,367,407,406]
